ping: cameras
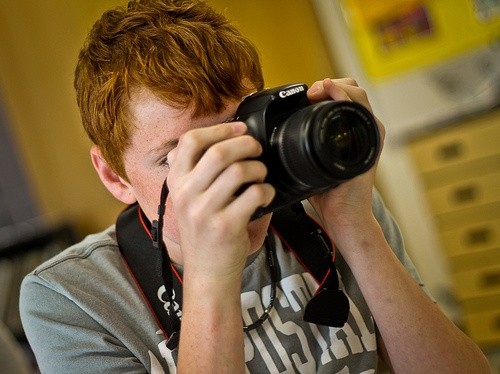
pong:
[222,81,381,223]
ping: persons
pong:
[18,1,494,374]
